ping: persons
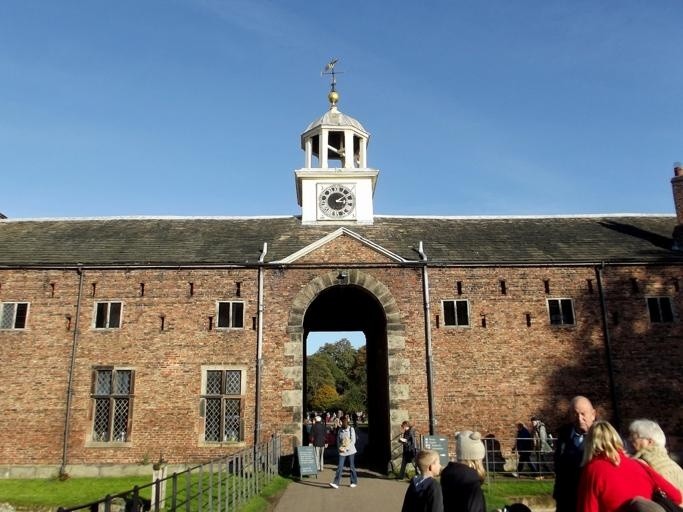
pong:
[508,420,538,478]
[302,412,342,442]
[528,417,556,480]
[395,421,419,481]
[309,415,329,472]
[439,429,487,511]
[627,419,683,507]
[582,418,683,511]
[551,395,598,511]
[327,415,358,489]
[401,448,444,512]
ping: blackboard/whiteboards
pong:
[297,446,318,475]
[422,434,448,469]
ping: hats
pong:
[455,430,485,459]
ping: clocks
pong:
[315,182,357,221]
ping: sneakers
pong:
[512,473,517,477]
[329,483,338,488]
[535,476,544,480]
[350,484,357,488]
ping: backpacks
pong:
[536,424,554,447]
